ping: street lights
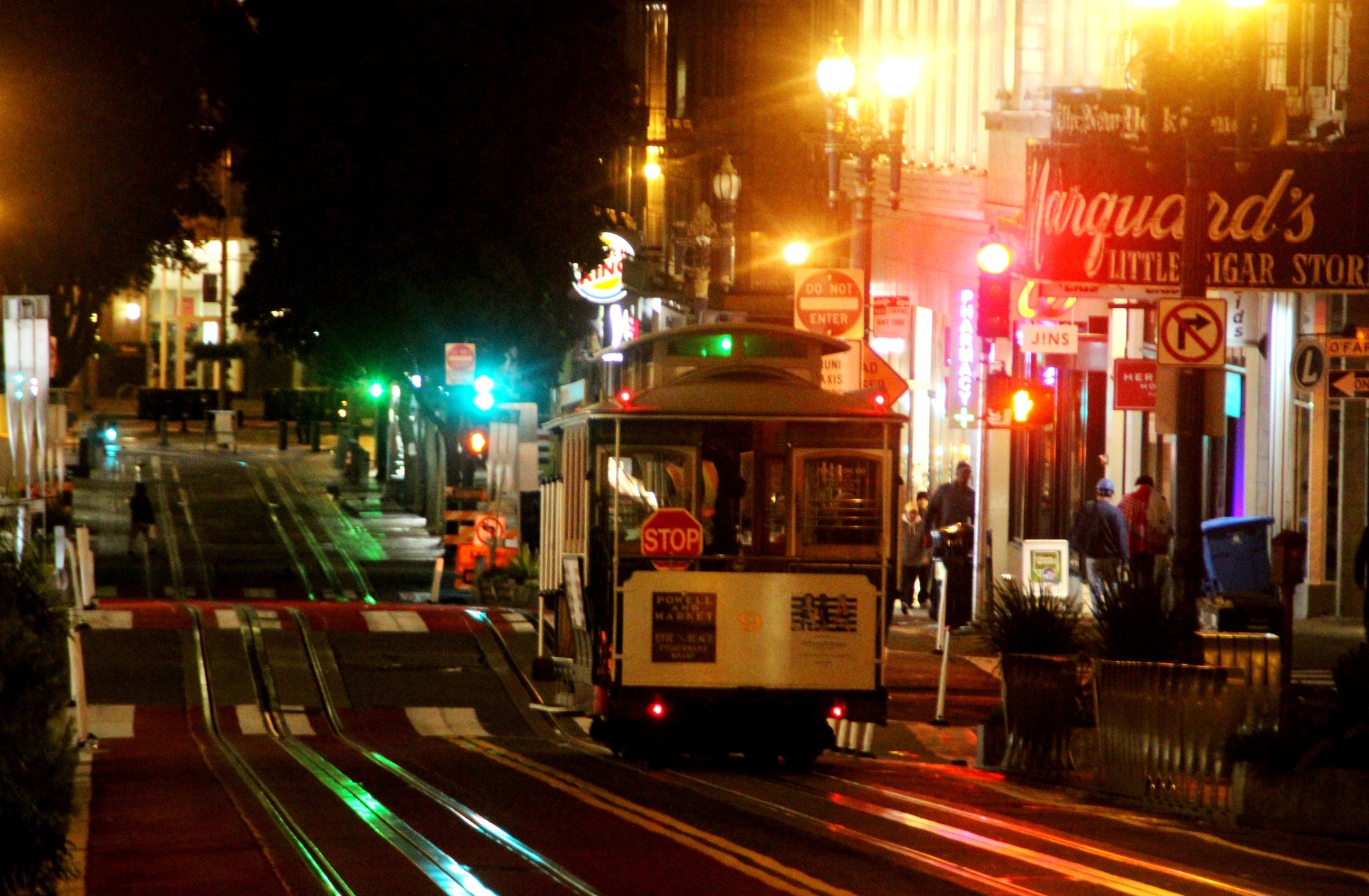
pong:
[1130,0,1284,662]
[710,146,743,290]
[817,51,919,342]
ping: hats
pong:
[904,500,919,513]
[956,461,971,472]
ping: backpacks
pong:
[1145,487,1173,535]
[1077,500,1104,558]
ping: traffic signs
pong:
[1327,370,1369,400]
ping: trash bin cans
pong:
[1213,588,1293,689]
[1202,516,1277,595]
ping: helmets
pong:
[1096,477,1114,492]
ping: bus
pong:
[531,322,913,778]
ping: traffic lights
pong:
[975,238,1011,339]
[367,379,386,400]
[1010,384,1054,425]
[469,371,496,418]
[465,429,489,455]
[105,420,117,442]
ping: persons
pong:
[898,500,925,611]
[1070,475,1131,630]
[1115,472,1175,592]
[122,479,168,564]
[915,491,936,605]
[920,460,975,624]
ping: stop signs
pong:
[444,342,476,386]
[792,264,865,340]
